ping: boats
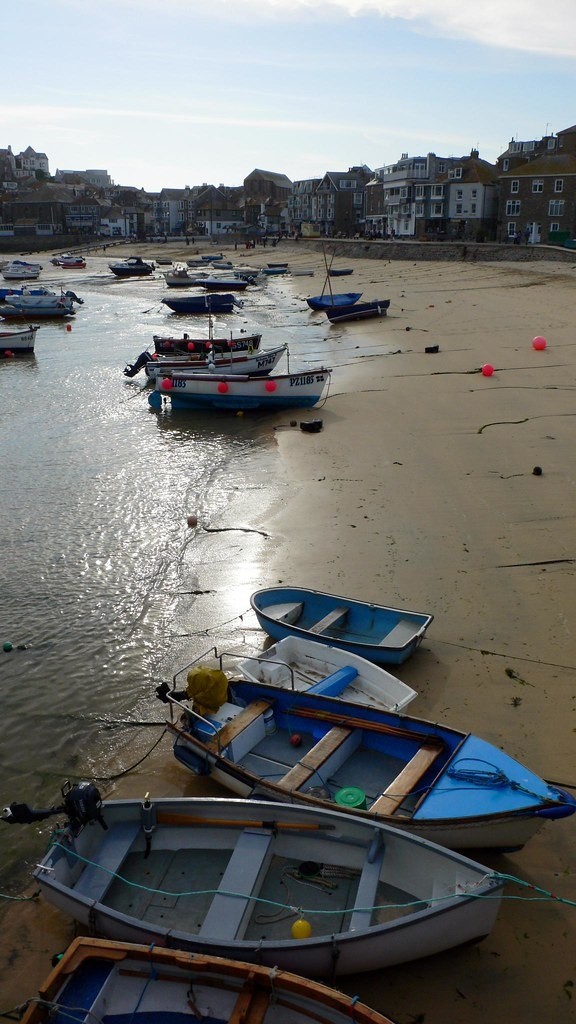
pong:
[0,320,40,354]
[0,298,76,320]
[153,299,262,359]
[161,293,244,315]
[249,585,435,669]
[305,247,363,310]
[155,646,576,857]
[328,268,354,277]
[123,342,291,385]
[19,934,396,1023]
[148,363,333,413]
[196,272,249,291]
[52,251,87,270]
[320,241,391,325]
[34,780,509,982]
[157,254,315,287]
[109,255,155,277]
[5,283,74,308]
[0,258,43,280]
[0,270,56,301]
[235,634,419,715]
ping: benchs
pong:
[72,820,139,902]
[368,745,443,814]
[208,694,275,753]
[277,725,362,794]
[228,981,273,1024]
[306,665,357,697]
[379,618,423,646]
[348,842,385,931]
[436,235,457,242]
[199,829,274,941]
[307,606,349,635]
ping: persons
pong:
[86,232,194,256]
[324,229,396,241]
[235,230,302,250]
[428,225,531,245]
